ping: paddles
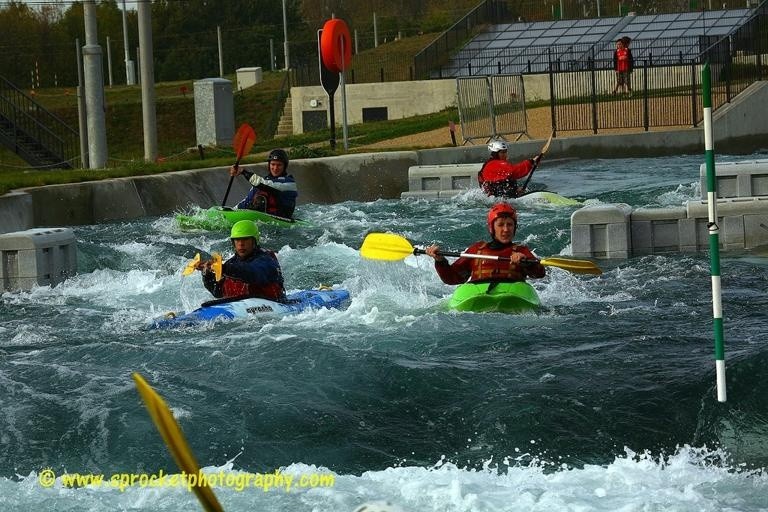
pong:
[224,125,255,209]
[184,253,224,285]
[522,129,556,195]
[359,232,601,277]
[133,374,226,511]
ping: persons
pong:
[229,150,299,217]
[612,36,633,98]
[477,141,541,198]
[201,220,283,302]
[425,201,545,283]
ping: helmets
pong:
[268,150,288,170]
[488,141,507,152]
[488,204,517,234]
[230,220,259,245]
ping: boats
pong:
[449,281,542,315]
[473,191,586,208]
[176,208,314,231]
[146,289,349,331]
